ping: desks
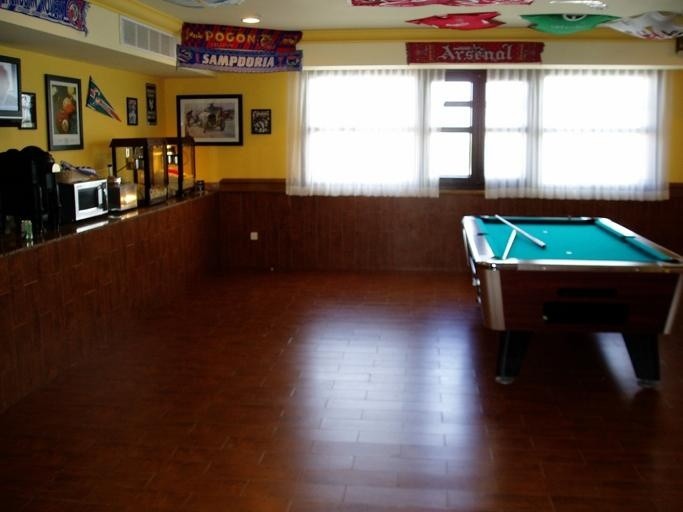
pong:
[460,215,683,384]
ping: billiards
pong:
[55,87,77,133]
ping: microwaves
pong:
[56,175,108,226]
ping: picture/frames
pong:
[0,55,22,128]
[145,83,158,126]
[176,94,243,146]
[126,97,138,125]
[44,73,84,151]
[251,109,272,134]
[18,92,37,129]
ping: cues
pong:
[502,229,516,260]
[495,214,547,248]
